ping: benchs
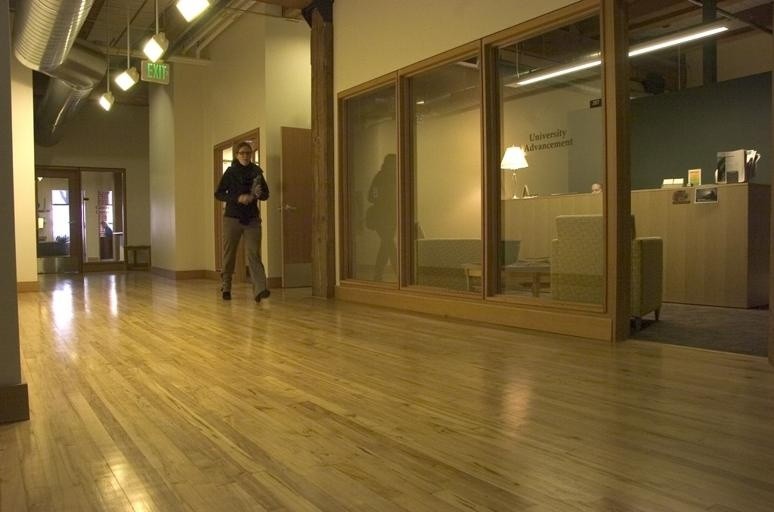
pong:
[412,237,521,294]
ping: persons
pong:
[367,154,396,282]
[101,221,112,237]
[214,142,271,303]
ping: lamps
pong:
[500,145,529,200]
[93,1,169,113]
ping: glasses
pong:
[238,151,251,155]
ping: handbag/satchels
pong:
[366,206,376,230]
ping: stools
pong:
[122,244,153,271]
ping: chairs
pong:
[548,214,665,332]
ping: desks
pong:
[459,259,553,299]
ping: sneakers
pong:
[223,292,230,300]
[255,290,270,302]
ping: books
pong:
[717,149,757,184]
